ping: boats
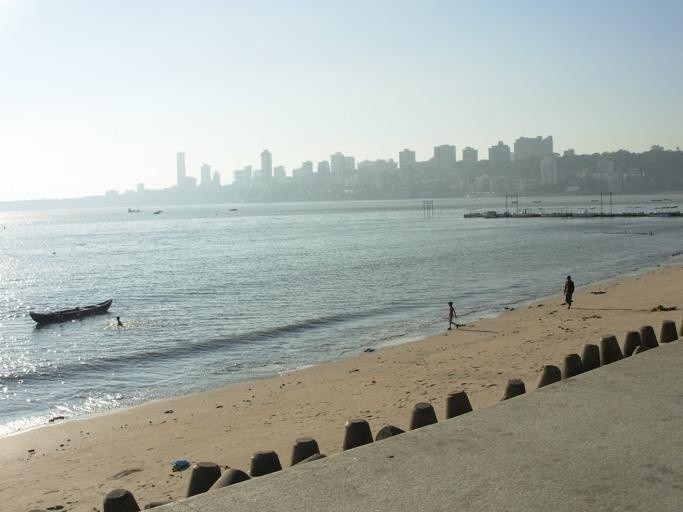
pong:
[29,299,114,322]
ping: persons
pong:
[563,275,574,309]
[116,316,121,326]
[446,301,458,330]
